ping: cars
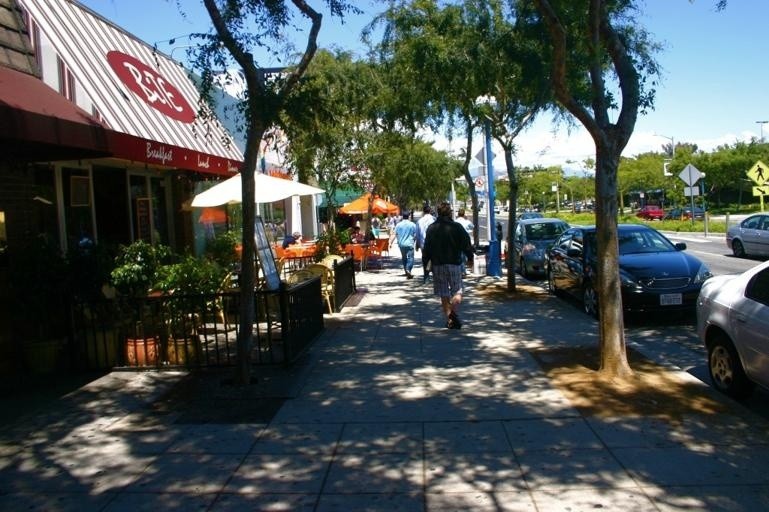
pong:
[503,219,571,281]
[636,205,664,221]
[545,222,713,326]
[665,205,706,221]
[693,259,769,403]
[516,213,545,221]
[723,212,769,259]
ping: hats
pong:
[437,202,454,217]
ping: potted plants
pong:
[109,238,232,369]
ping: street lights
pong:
[652,133,676,160]
[756,119,768,144]
[698,172,706,196]
[475,91,506,277]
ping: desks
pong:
[286,240,321,273]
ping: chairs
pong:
[259,256,337,322]
[345,238,390,271]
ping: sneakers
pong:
[446,309,457,328]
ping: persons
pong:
[283,232,301,248]
[350,204,474,284]
[422,202,473,329]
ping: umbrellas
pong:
[189,169,326,214]
[338,191,400,215]
